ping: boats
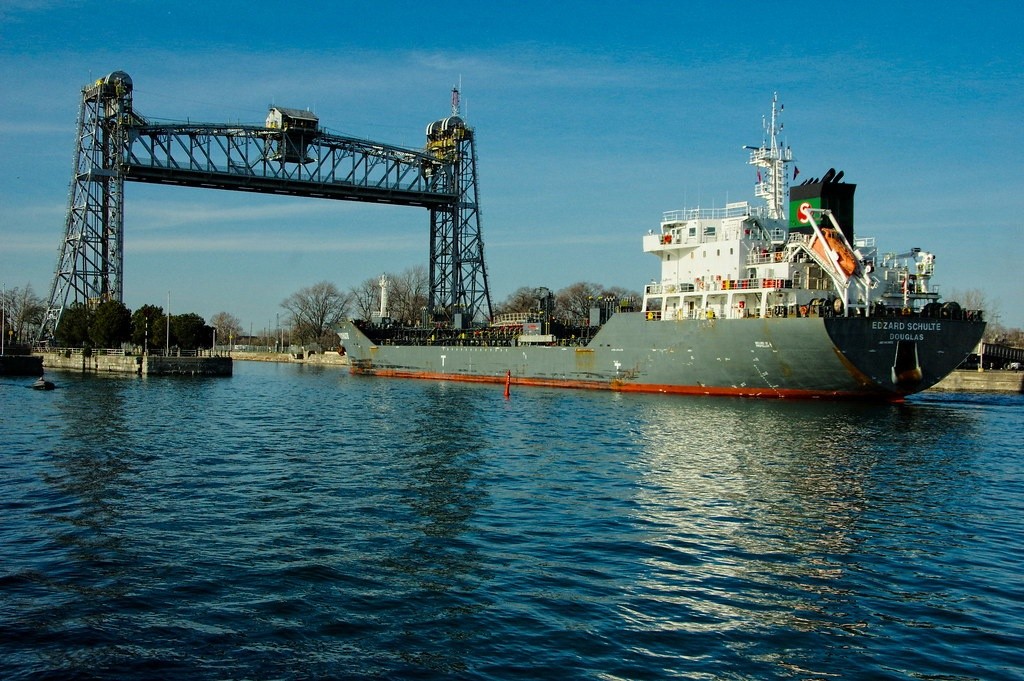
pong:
[322,91,988,403]
[33,380,55,391]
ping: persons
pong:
[823,295,886,318]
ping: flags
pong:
[793,166,799,181]
